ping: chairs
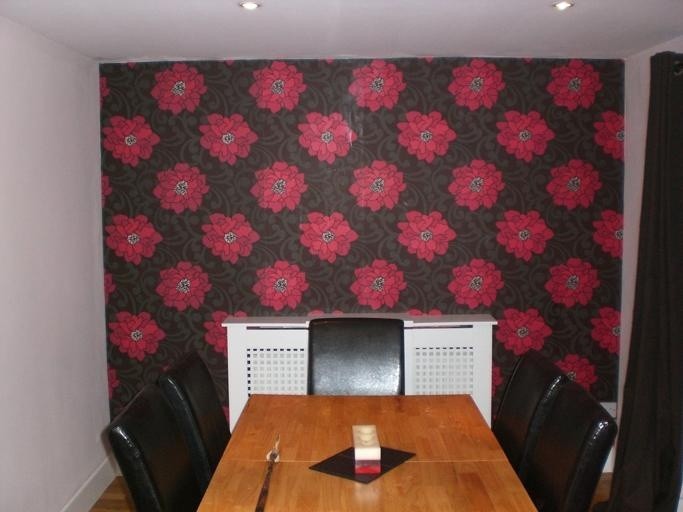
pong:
[489,348,617,511]
[107,350,231,511]
[307,317,405,395]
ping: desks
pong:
[194,394,540,512]
